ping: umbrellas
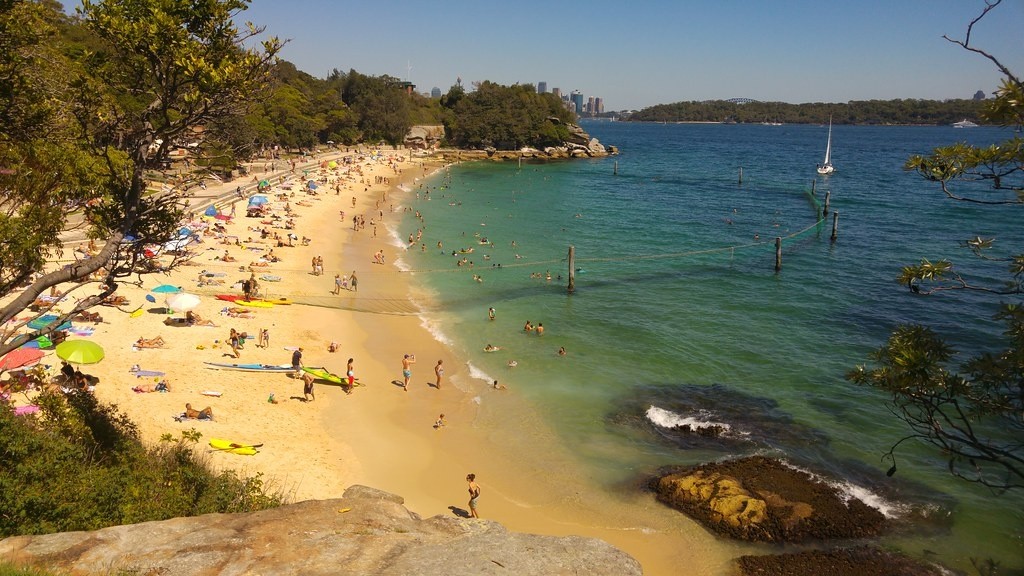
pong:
[137,247,160,259]
[10,332,54,350]
[165,293,201,323]
[26,314,72,341]
[0,346,46,380]
[56,339,105,374]
[151,283,180,314]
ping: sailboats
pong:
[817,114,836,175]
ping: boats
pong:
[214,294,292,305]
[209,437,258,455]
[234,299,273,307]
[203,360,323,373]
[300,366,360,386]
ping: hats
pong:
[298,370,305,377]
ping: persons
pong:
[466,474,481,518]
[185,402,215,422]
[0,135,591,410]
[724,198,810,240]
[435,413,447,430]
